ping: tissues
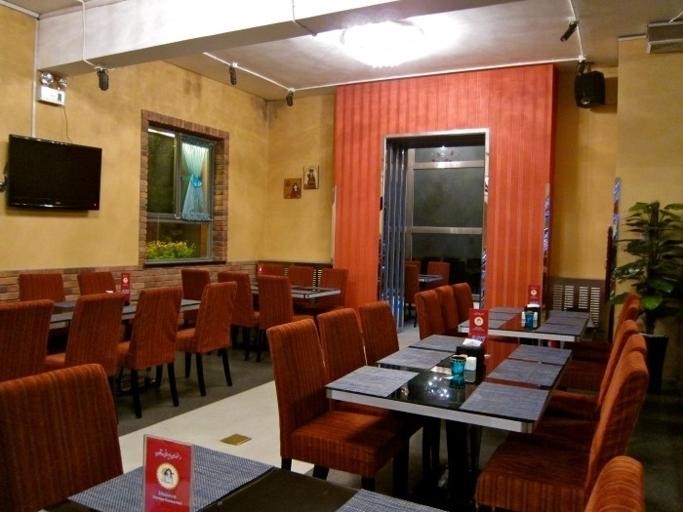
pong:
[455,336,485,368]
[525,301,541,327]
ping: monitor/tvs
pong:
[6,133,104,213]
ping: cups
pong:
[449,356,465,376]
[448,374,465,390]
[523,311,534,323]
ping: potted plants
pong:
[606,201,682,401]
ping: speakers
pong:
[573,69,607,110]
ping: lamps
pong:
[229,63,238,86]
[94,67,111,91]
[559,19,580,43]
[283,87,295,108]
[34,69,67,108]
